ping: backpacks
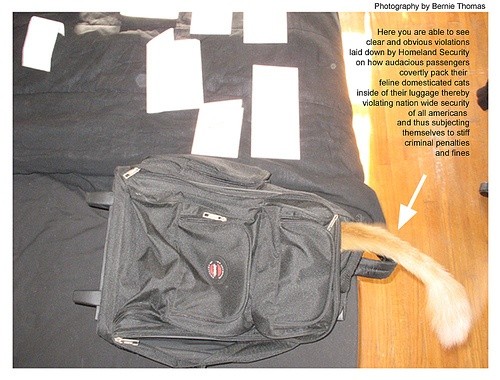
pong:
[71,155,397,368]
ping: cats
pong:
[340,220,473,354]
